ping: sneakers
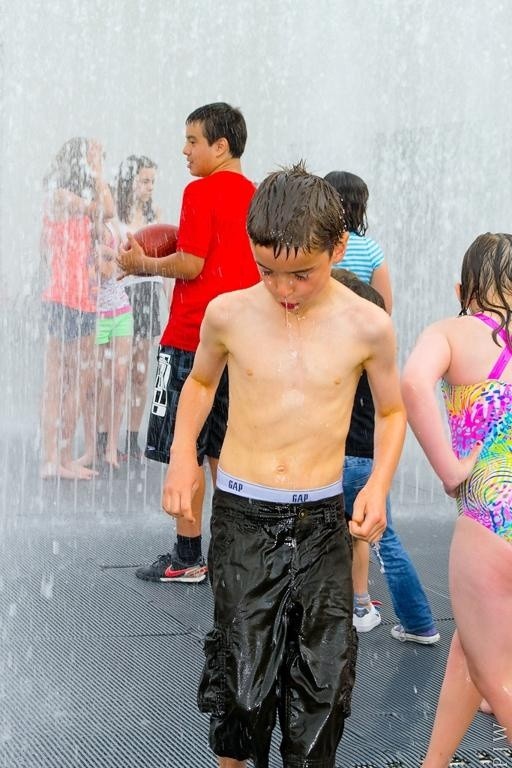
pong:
[352,602,381,633]
[135,543,209,584]
[392,624,440,645]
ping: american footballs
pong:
[126,223,179,276]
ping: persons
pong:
[323,171,394,634]
[329,268,440,645]
[39,136,173,477]
[401,230,512,768]
[161,159,408,768]
[116,102,261,584]
